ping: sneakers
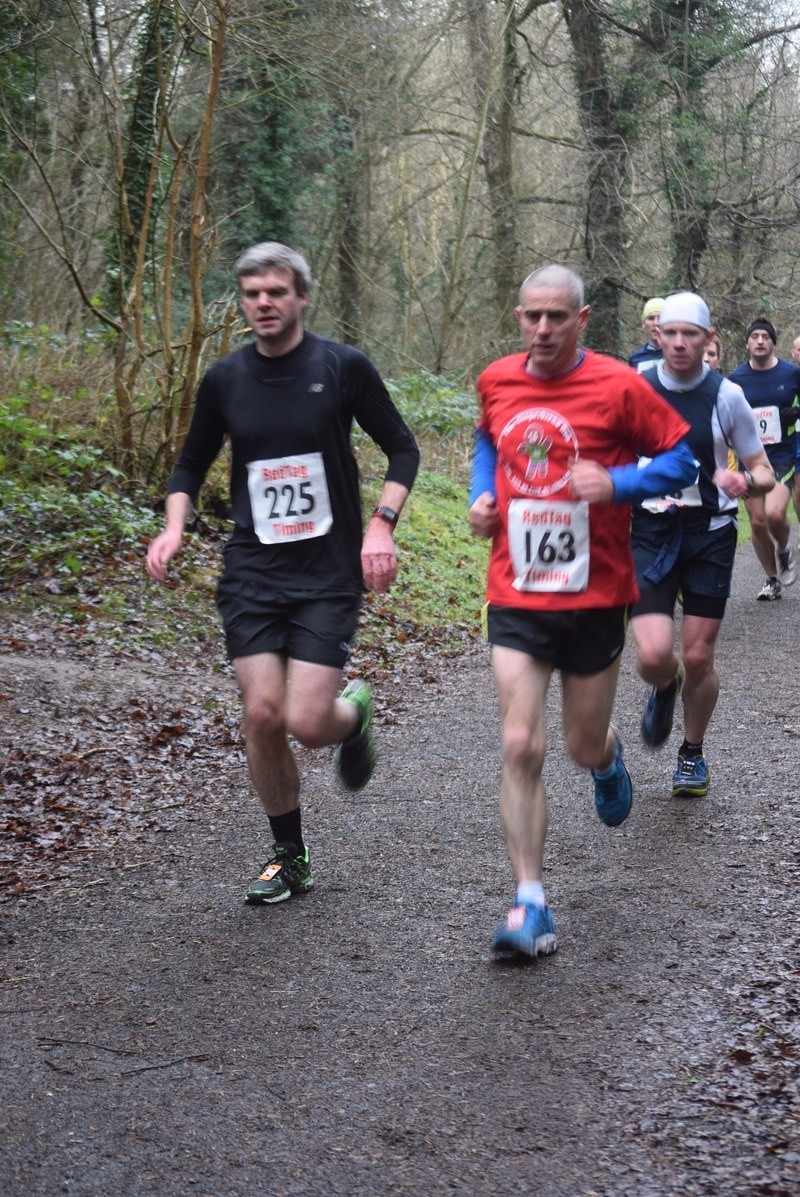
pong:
[672,753,710,797]
[333,680,375,792]
[493,898,556,960]
[590,724,633,829]
[245,841,314,904]
[757,577,782,600]
[776,545,797,584]
[640,666,685,748]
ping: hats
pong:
[659,292,711,333]
[641,297,665,321]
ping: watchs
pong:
[739,470,756,500]
[372,506,398,529]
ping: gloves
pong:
[779,405,800,428]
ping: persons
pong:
[702,336,722,370]
[629,297,665,374]
[147,245,422,907]
[628,291,777,796]
[727,317,800,601]
[468,265,699,958]
